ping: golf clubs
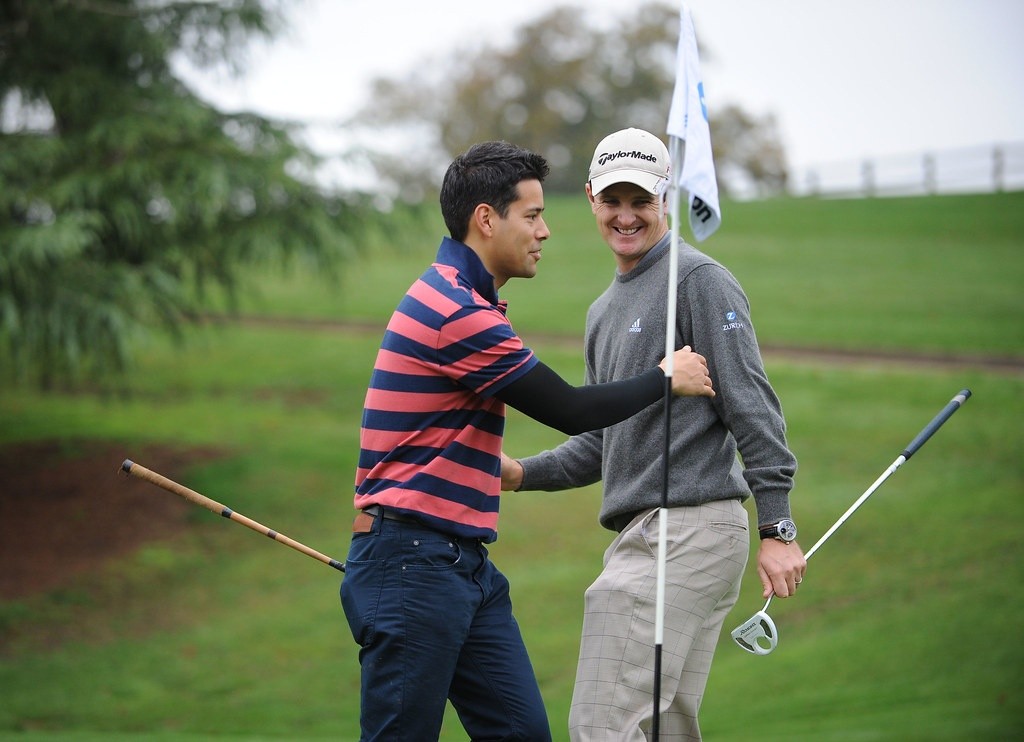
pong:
[121,458,346,574]
[730,388,974,657]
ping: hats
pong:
[587,127,673,197]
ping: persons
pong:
[340,140,716,742]
[500,126,807,742]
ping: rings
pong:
[795,577,803,583]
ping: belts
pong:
[361,504,423,526]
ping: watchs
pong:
[759,518,797,545]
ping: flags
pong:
[666,5,722,245]
